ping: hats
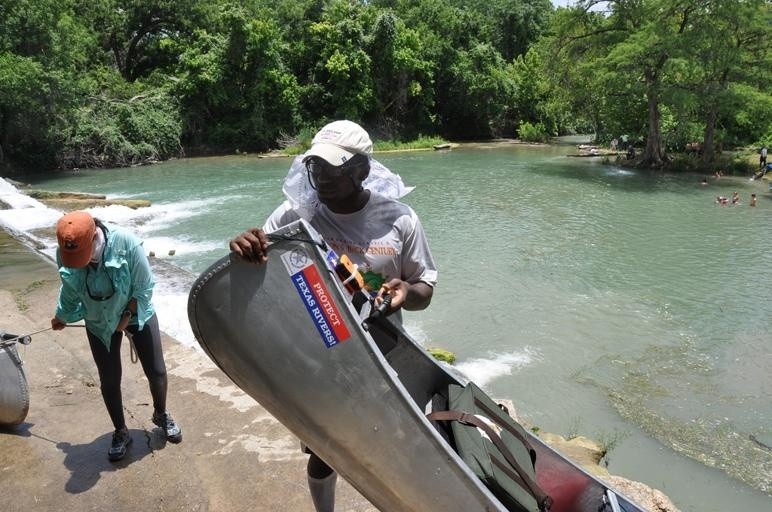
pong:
[301,119,375,168]
[56,209,97,270]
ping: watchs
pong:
[123,309,133,320]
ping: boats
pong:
[0,334,35,428]
[432,143,455,150]
[187,218,647,512]
[565,142,623,158]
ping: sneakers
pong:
[150,412,182,441]
[108,427,132,460]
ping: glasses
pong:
[306,158,365,176]
[85,269,116,302]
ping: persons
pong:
[227,116,442,511]
[49,208,183,464]
[684,139,767,210]
[608,132,647,161]
